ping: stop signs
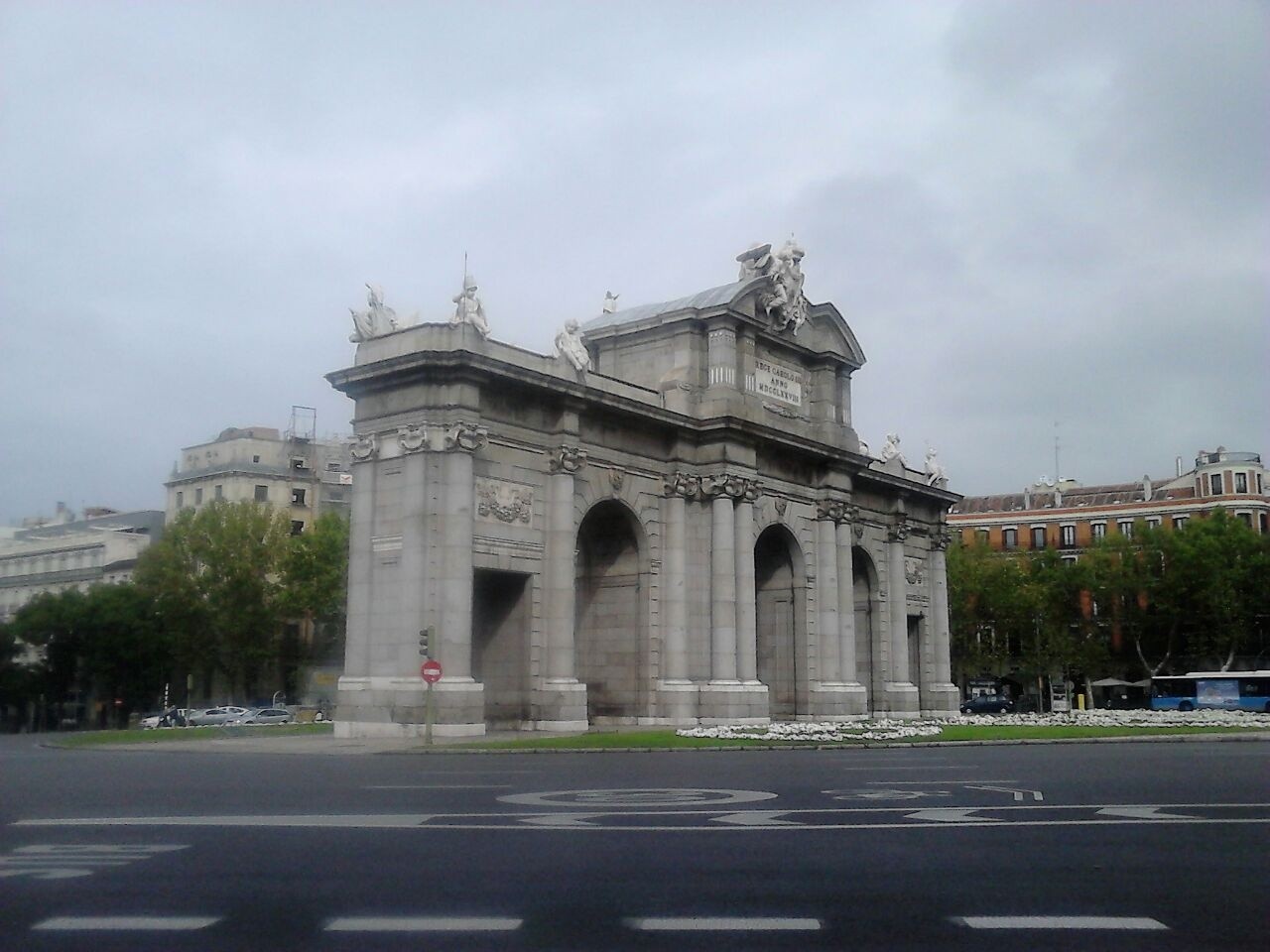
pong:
[421,661,443,683]
[114,698,124,708]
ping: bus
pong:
[1151,670,1270,711]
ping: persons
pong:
[766,235,805,316]
[350,286,398,343]
[452,276,492,336]
[555,318,593,371]
[882,432,909,467]
[924,445,943,486]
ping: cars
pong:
[139,706,249,726]
[225,708,293,724]
[960,694,1016,713]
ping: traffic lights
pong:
[419,626,436,659]
